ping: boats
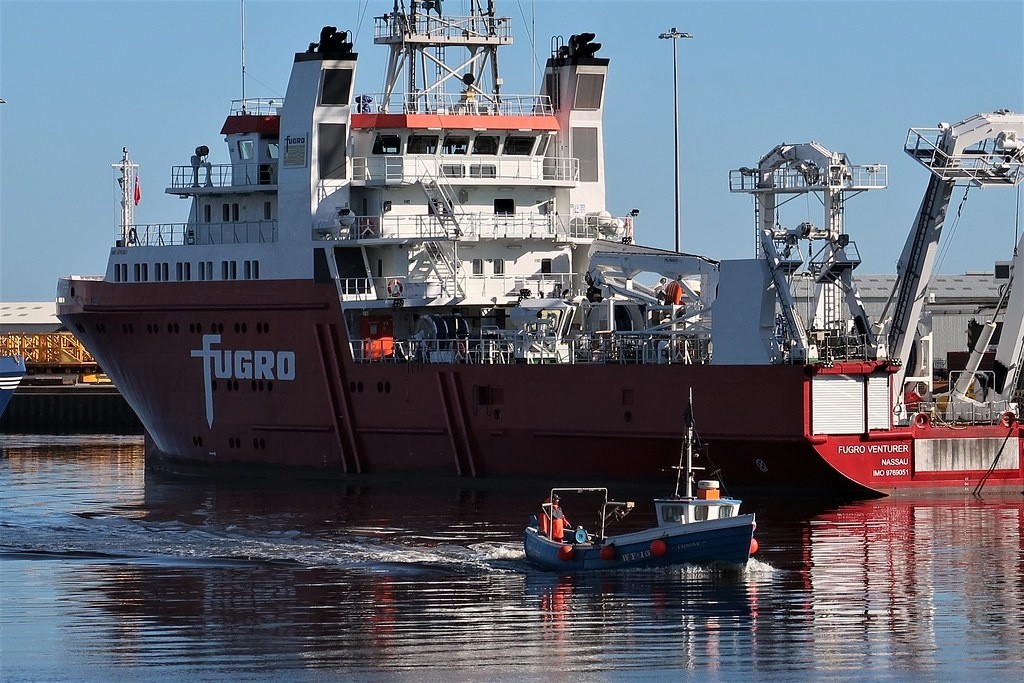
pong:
[55,0,1024,497]
[0,344,26,418]
[522,387,756,576]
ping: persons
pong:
[540,494,571,543]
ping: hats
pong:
[552,494,561,500]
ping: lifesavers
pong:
[388,279,403,298]
[361,218,376,236]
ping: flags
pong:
[133,174,142,206]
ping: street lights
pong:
[658,27,693,253]
